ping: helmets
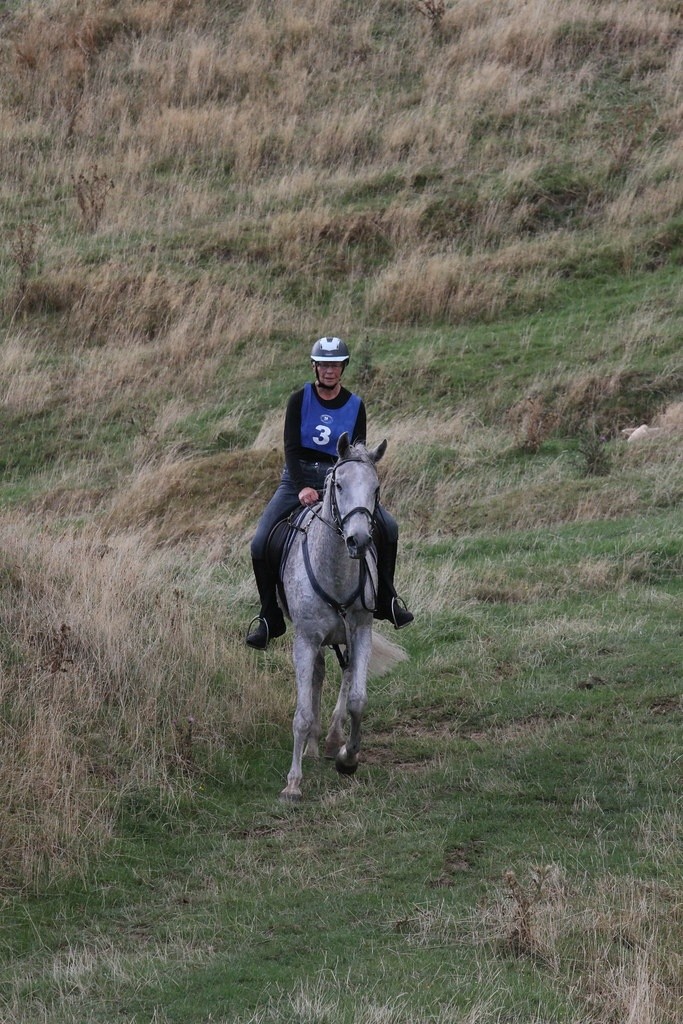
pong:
[308,336,351,363]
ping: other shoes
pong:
[247,604,289,646]
[374,594,416,627]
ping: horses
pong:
[271,431,409,804]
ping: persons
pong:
[246,337,414,647]
